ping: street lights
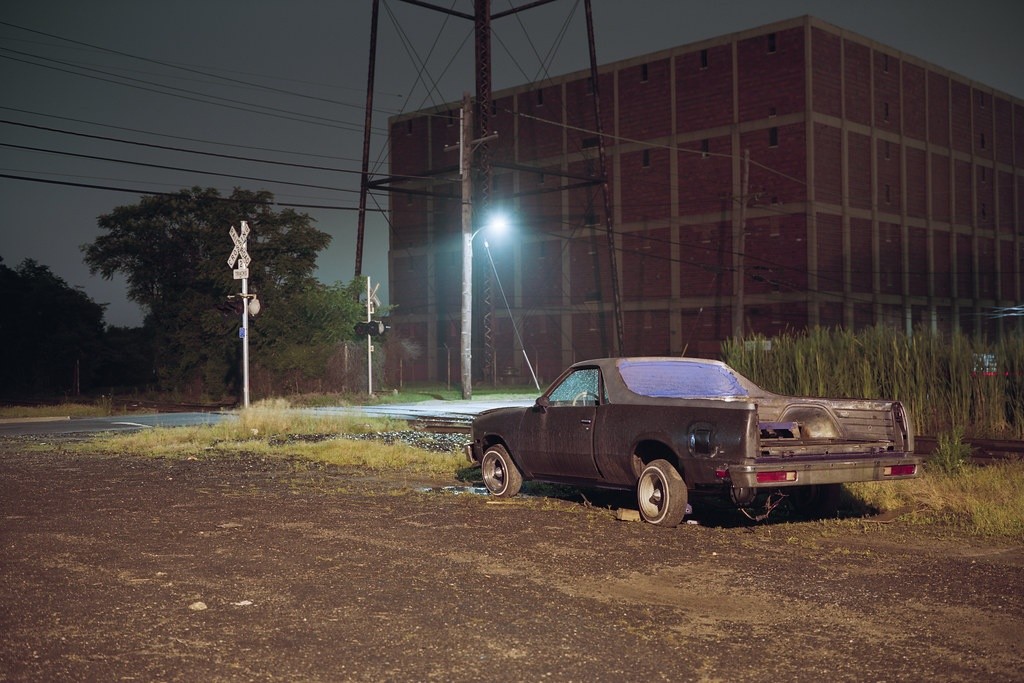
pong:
[459,214,512,401]
[227,220,262,408]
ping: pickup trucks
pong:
[464,355,919,526]
